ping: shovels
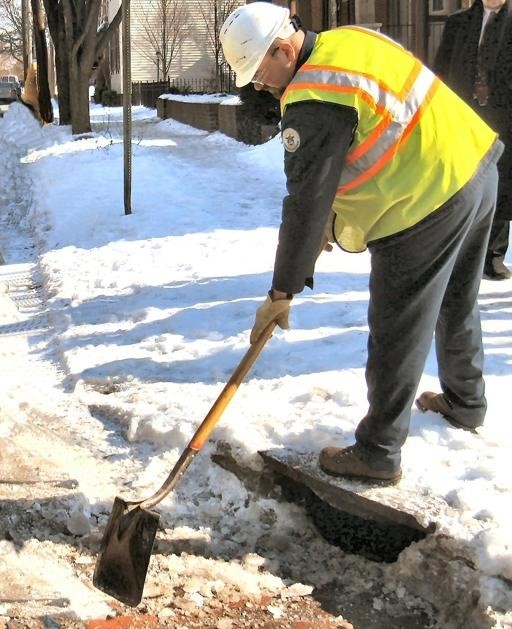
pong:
[92,320,277,607]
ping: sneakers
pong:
[416,390,478,434]
[318,446,403,486]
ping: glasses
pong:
[251,46,278,86]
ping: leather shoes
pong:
[484,260,512,280]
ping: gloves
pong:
[321,210,335,252]
[250,292,293,344]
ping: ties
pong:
[476,9,497,106]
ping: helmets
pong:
[219,1,290,87]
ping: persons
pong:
[430,0,512,282]
[218,2,504,485]
